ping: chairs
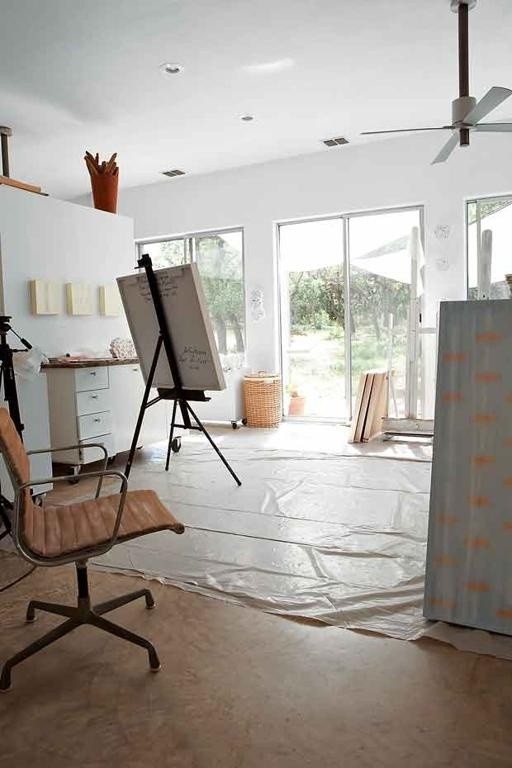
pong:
[0,408,185,693]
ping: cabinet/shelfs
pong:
[0,370,53,507]
[41,359,189,484]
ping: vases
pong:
[289,397,305,416]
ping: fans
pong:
[359,0,512,165]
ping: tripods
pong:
[0,315,33,542]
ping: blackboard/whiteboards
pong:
[116,262,226,391]
